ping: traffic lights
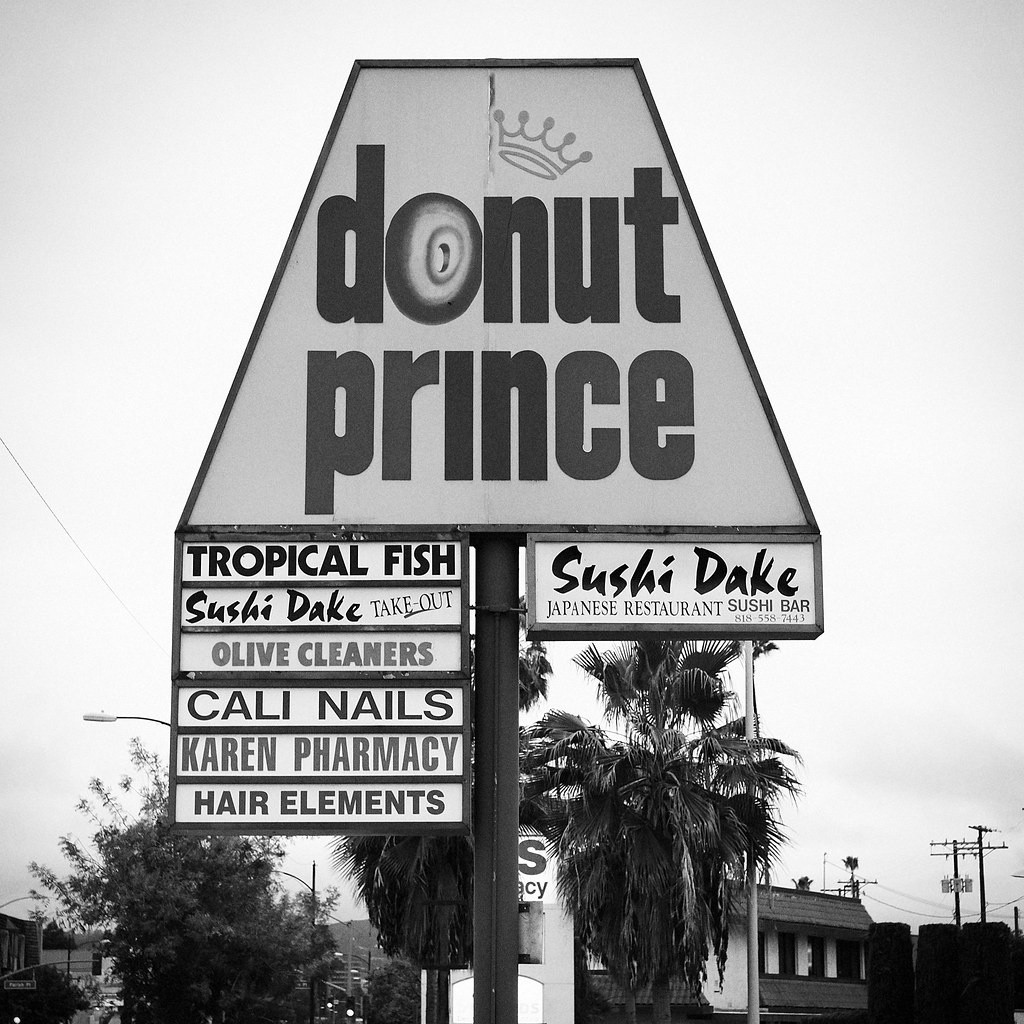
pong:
[327,997,334,1010]
[345,995,357,1020]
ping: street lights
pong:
[334,949,374,974]
[83,708,175,735]
